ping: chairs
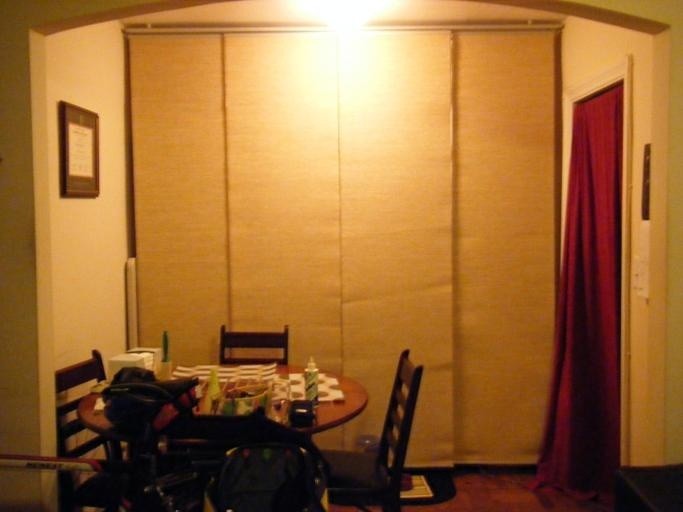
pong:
[52,325,369,512]
[320,347,422,510]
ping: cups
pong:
[264,380,290,426]
[291,400,314,428]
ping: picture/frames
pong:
[57,99,99,197]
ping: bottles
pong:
[203,368,222,414]
[305,357,319,409]
